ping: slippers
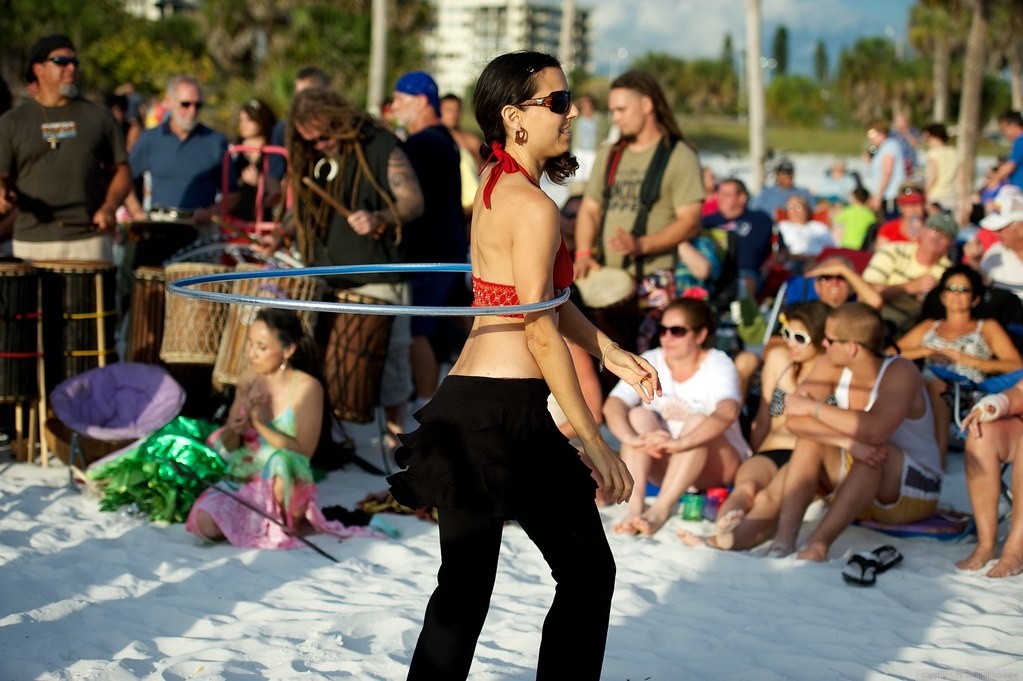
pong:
[841,546,904,587]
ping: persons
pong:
[0,33,1022,574]
[384,49,661,681]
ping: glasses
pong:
[47,56,80,68]
[518,89,573,113]
[823,332,848,346]
[307,133,331,147]
[818,274,845,281]
[179,100,203,109]
[782,327,812,349]
[943,286,972,294]
[655,325,695,338]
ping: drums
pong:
[160,262,234,363]
[320,286,396,423]
[571,267,641,401]
[32,259,120,410]
[211,262,329,392]
[125,266,225,421]
[0,263,38,403]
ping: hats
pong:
[898,188,925,203]
[396,70,442,118]
[925,213,957,239]
[979,193,1023,231]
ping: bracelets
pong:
[573,250,590,257]
[373,211,386,234]
[814,402,822,419]
[599,342,619,373]
